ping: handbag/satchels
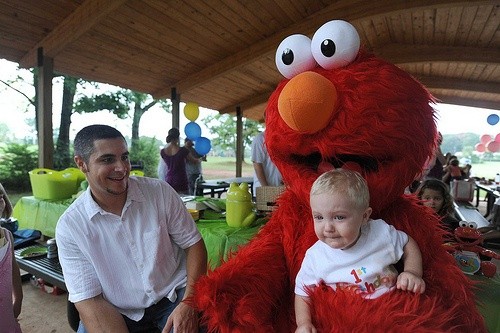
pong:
[450,178,474,202]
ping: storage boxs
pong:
[28,168,144,200]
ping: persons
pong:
[250,107,284,202]
[55,125,214,333]
[160,128,207,196]
[404,132,500,247]
[0,183,23,333]
[295,168,426,333]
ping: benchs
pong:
[13,239,69,292]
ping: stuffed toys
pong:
[182,19,488,333]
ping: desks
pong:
[475,180,500,215]
[198,176,254,200]
[20,193,500,333]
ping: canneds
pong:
[46,239,58,258]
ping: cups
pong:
[225,182,256,228]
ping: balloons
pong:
[474,133,500,153]
[184,102,211,156]
[487,114,500,125]
